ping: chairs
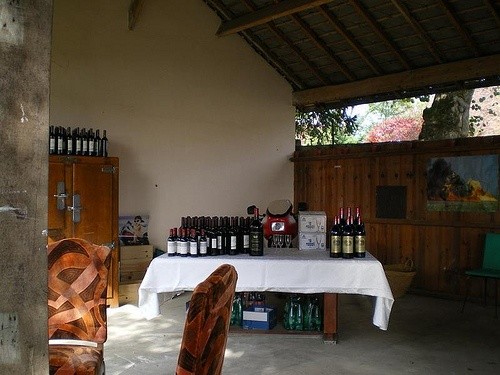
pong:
[462,232,500,319]
[48,237,114,375]
[175,264,238,375]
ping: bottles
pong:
[229,292,324,331]
[329,207,366,259]
[48,124,109,157]
[166,208,263,256]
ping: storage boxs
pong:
[298,211,330,251]
[120,245,153,281]
[242,304,279,330]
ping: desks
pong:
[138,248,395,345]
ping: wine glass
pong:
[273,234,291,254]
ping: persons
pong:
[124,216,147,243]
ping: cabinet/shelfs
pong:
[48,154,119,309]
